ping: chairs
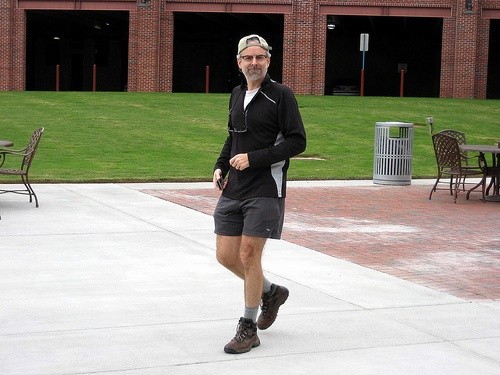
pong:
[0,127,45,207]
[429,129,500,203]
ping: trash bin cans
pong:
[373,122,413,185]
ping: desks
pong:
[458,145,500,202]
[0,140,14,167]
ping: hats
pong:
[238,35,272,54]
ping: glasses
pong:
[226,108,247,132]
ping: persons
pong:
[213,35,307,354]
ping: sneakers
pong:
[224,317,260,354]
[257,284,289,330]
